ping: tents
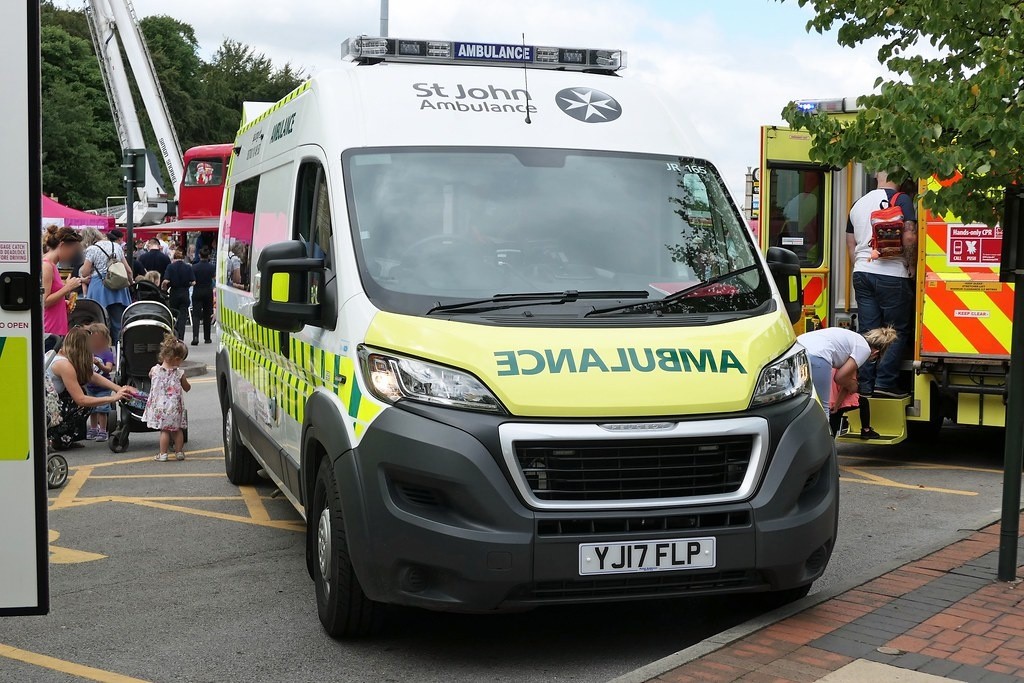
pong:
[41,190,116,233]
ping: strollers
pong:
[108,300,189,452]
[130,279,181,340]
[68,298,112,340]
[44,334,69,488]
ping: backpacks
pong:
[91,242,128,289]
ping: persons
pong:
[44,323,134,450]
[778,176,823,268]
[189,249,216,345]
[207,240,218,283]
[140,333,191,461]
[41,222,82,349]
[86,324,114,442]
[159,251,196,346]
[226,237,252,293]
[65,230,197,299]
[791,325,895,426]
[79,226,133,345]
[846,169,920,400]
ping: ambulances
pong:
[213,34,842,635]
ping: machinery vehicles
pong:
[80,0,235,256]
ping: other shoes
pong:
[191,337,198,345]
[205,340,212,344]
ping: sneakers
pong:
[87,428,109,441]
[154,452,168,461]
[175,451,185,461]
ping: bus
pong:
[758,88,1014,449]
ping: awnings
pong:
[132,217,221,233]
